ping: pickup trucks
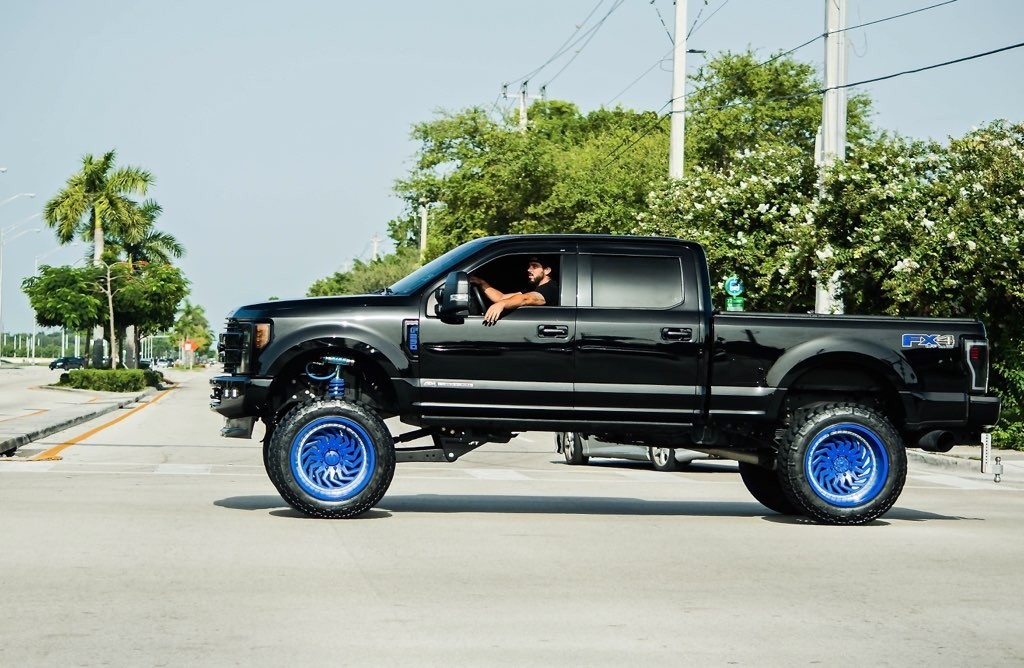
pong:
[208,233,1005,528]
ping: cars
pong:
[551,427,736,472]
[139,355,175,368]
[49,356,110,371]
[197,355,217,368]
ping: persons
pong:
[468,256,557,323]
